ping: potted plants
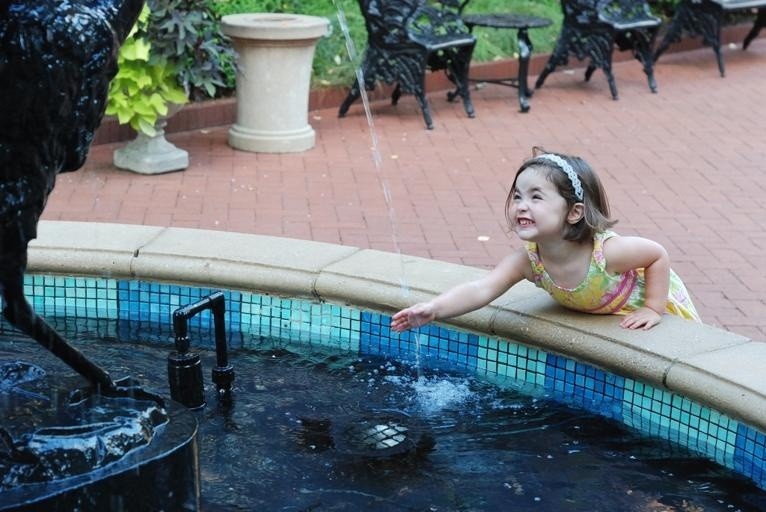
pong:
[102,2,238,177]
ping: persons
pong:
[388,151,703,335]
